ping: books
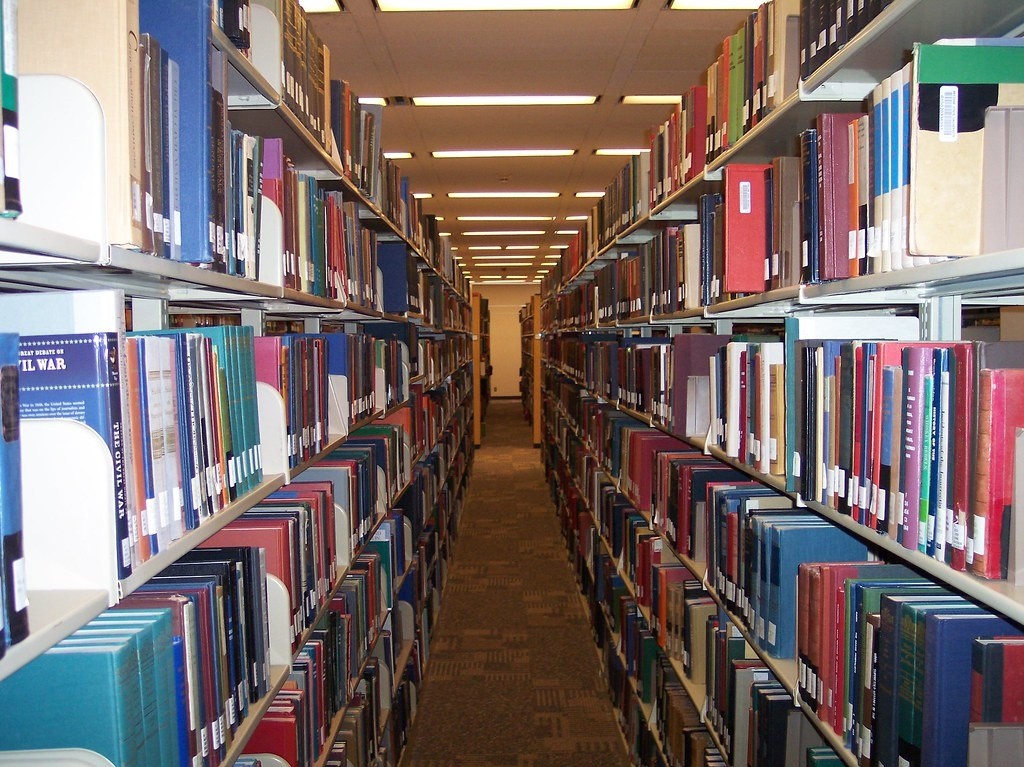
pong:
[518,0,1024,767]
[0,0,494,767]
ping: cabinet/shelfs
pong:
[0,1,473,767]
[473,292,492,449]
[541,0,1024,767]
[519,294,541,448]
